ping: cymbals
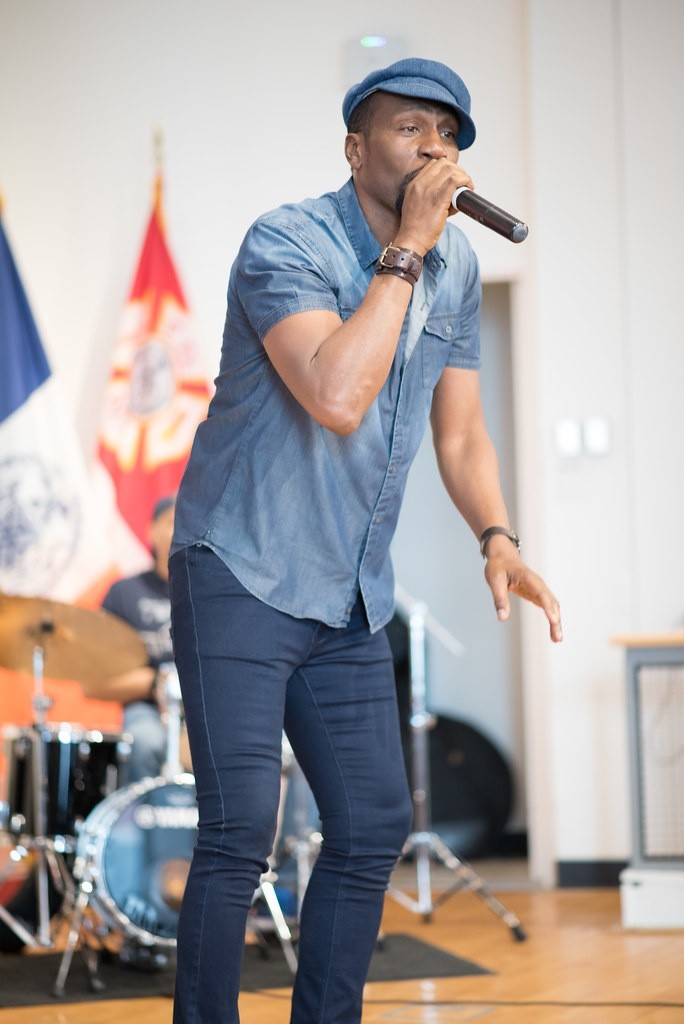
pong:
[0,594,148,682]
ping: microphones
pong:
[451,185,529,243]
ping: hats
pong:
[342,58,476,152]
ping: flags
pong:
[1,196,57,421]
[96,202,217,553]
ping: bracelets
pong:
[150,669,160,706]
[374,242,426,283]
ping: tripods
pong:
[388,588,529,946]
[0,628,118,969]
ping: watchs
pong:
[480,526,522,560]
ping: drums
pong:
[76,772,196,948]
[3,722,124,853]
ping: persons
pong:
[86,499,308,936]
[168,57,562,1024]
